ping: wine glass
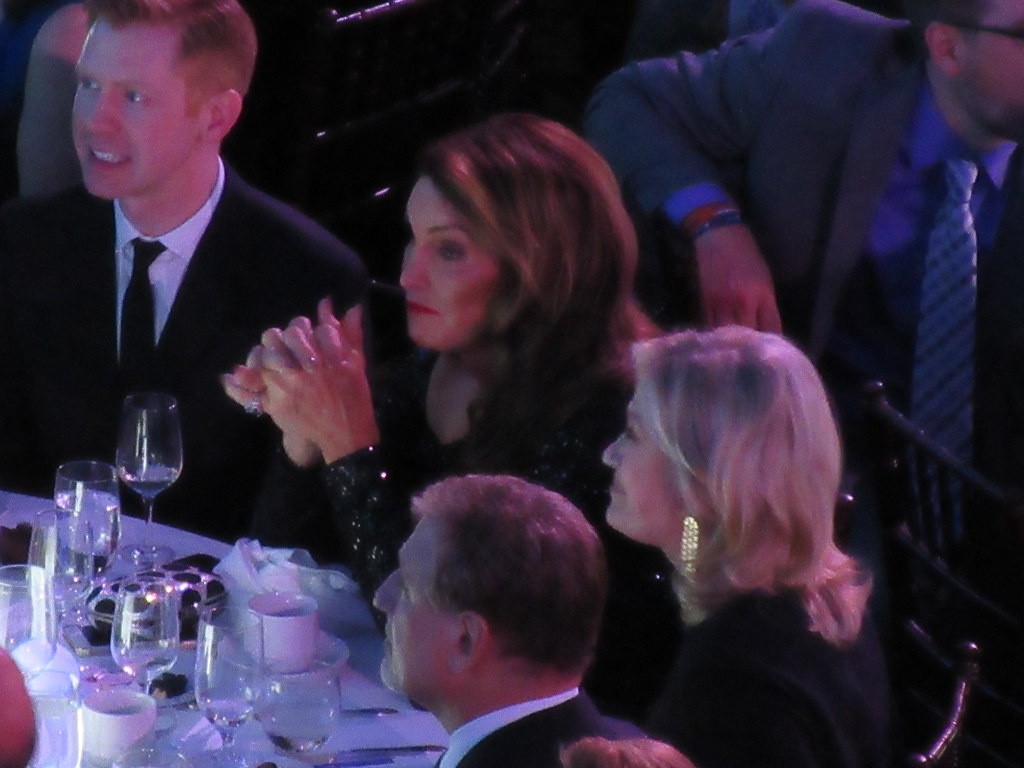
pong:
[110,576,180,735]
[116,392,184,565]
[194,607,267,768]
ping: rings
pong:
[241,393,265,417]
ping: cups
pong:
[79,689,157,768]
[22,670,81,768]
[54,461,121,577]
[258,659,342,755]
[0,565,58,680]
[26,508,94,615]
[112,749,194,768]
[248,593,319,672]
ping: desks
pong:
[0,490,450,768]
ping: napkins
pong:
[212,535,360,620]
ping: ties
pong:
[119,237,168,394]
[908,158,979,548]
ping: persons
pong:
[371,473,650,768]
[557,735,697,768]
[581,0,1024,593]
[0,0,377,553]
[219,111,676,712]
[0,1,100,201]
[601,324,896,768]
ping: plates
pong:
[217,630,350,673]
[77,648,213,708]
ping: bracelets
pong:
[685,208,744,244]
